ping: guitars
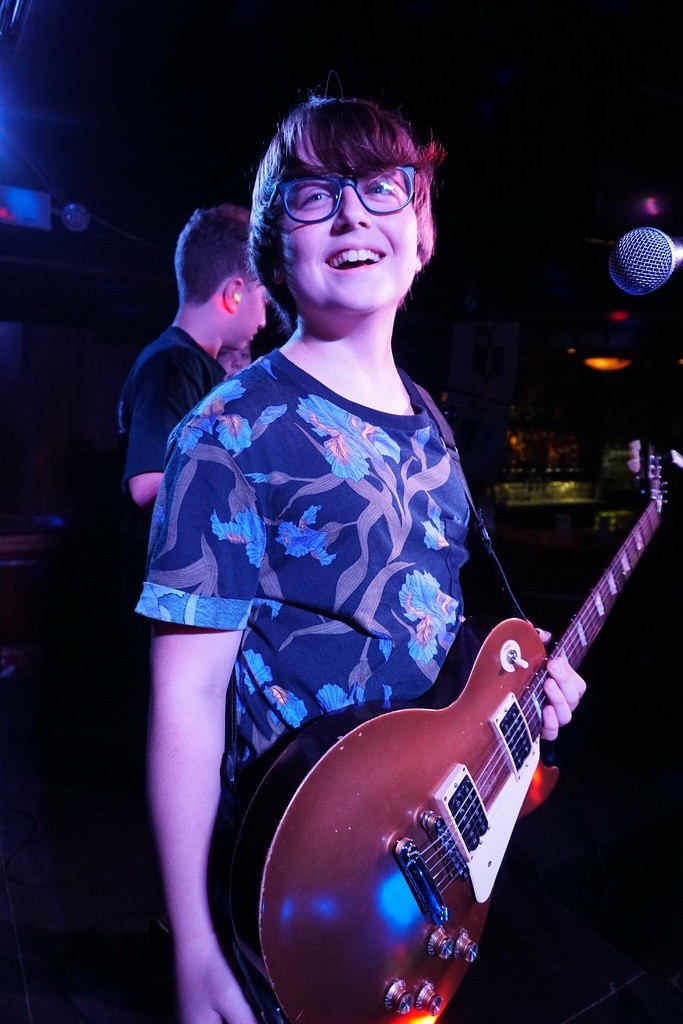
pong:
[216,436,683,1024]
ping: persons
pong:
[118,204,272,939]
[135,94,586,1024]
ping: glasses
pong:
[269,165,416,222]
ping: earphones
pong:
[233,293,240,303]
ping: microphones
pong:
[610,227,683,295]
